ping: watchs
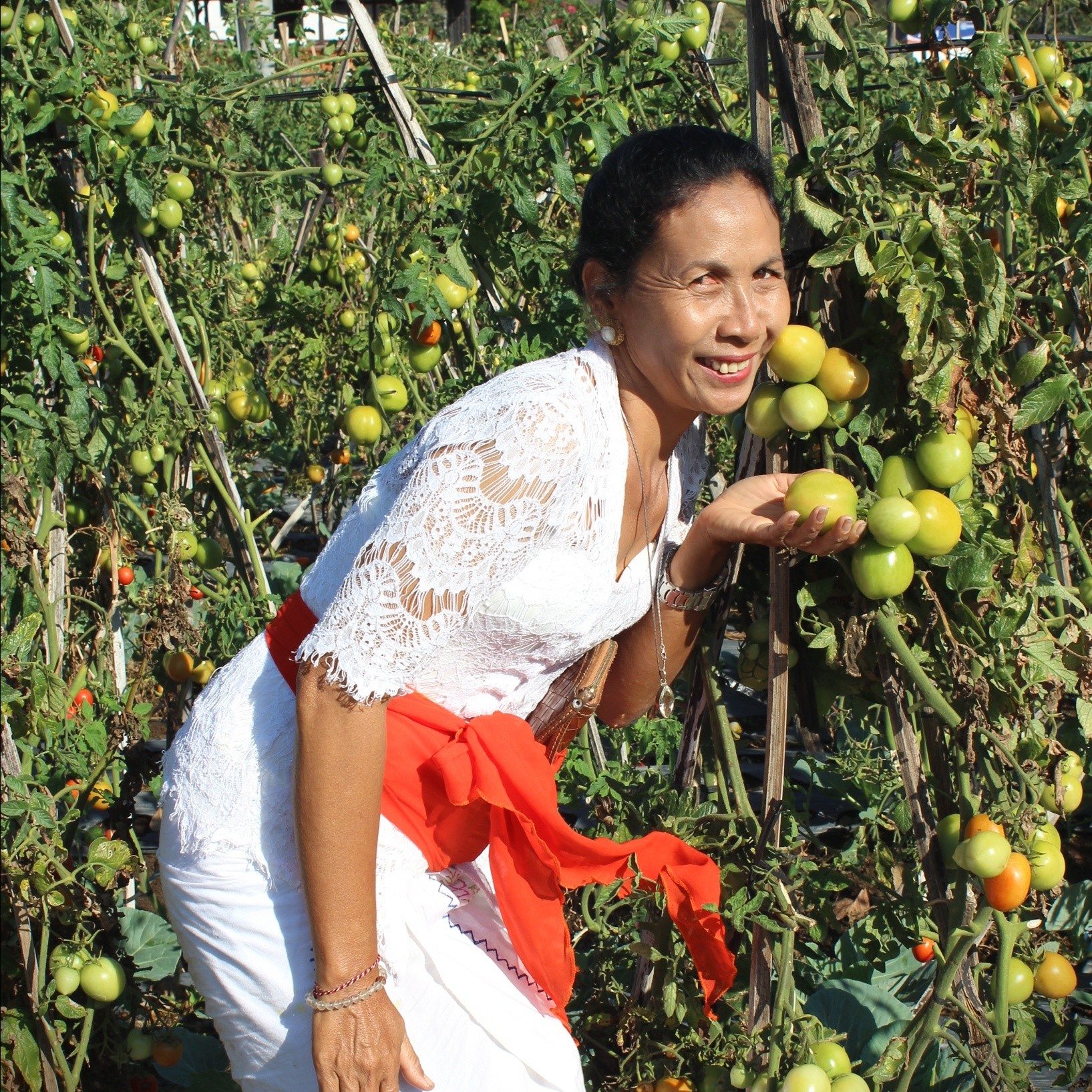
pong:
[655,544,733,610]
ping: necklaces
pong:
[620,405,676,723]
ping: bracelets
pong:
[313,954,381,996]
[305,968,387,1010]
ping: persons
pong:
[156,122,869,1092]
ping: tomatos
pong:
[1,0,1087,1092]
